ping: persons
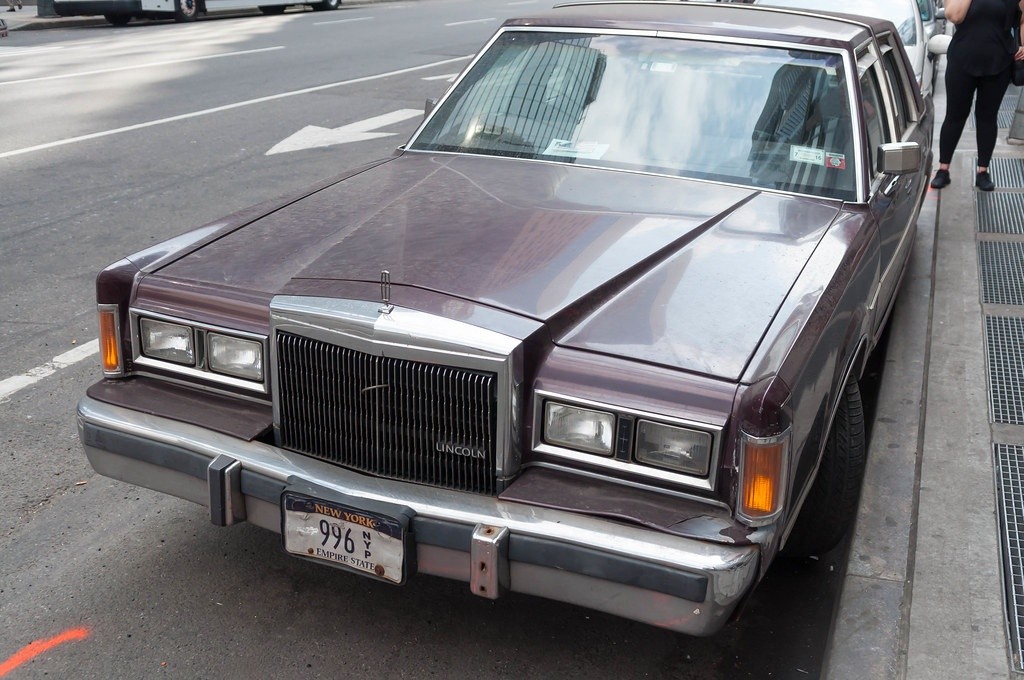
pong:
[930,0,1024,190]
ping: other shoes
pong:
[931,169,950,189]
[976,171,994,191]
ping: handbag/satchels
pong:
[1013,49,1024,86]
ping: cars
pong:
[754,0,952,100]
[53,1,342,25]
[77,0,939,638]
